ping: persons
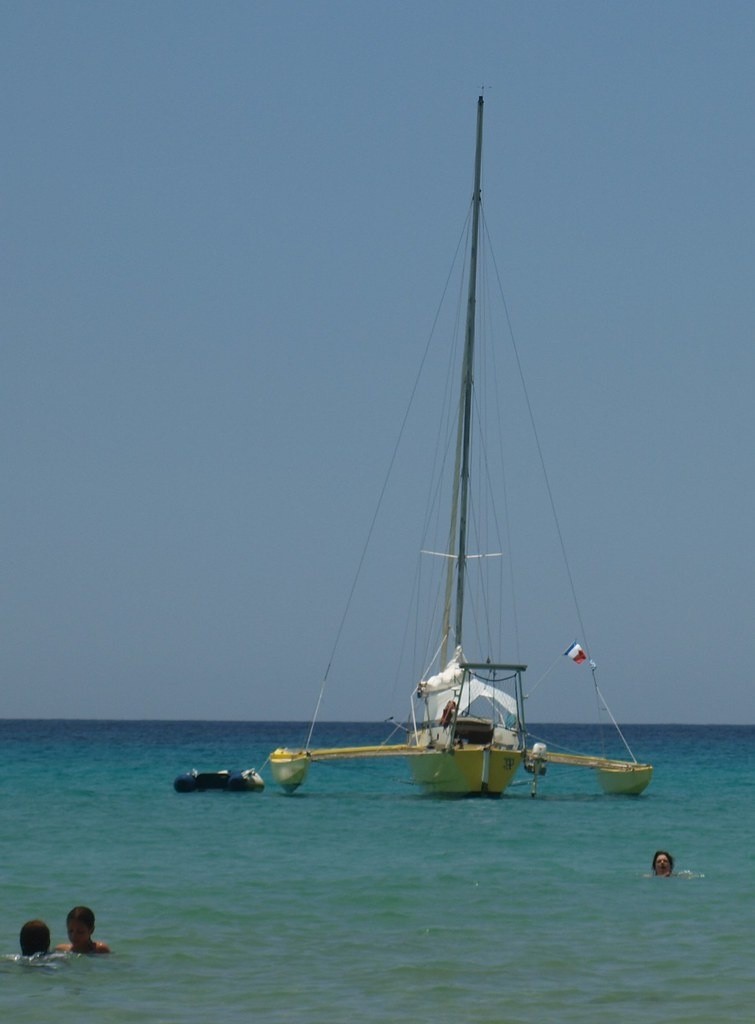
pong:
[20,920,50,956]
[55,906,109,953]
[651,851,674,876]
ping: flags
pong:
[563,640,587,665]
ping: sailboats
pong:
[266,89,657,805]
